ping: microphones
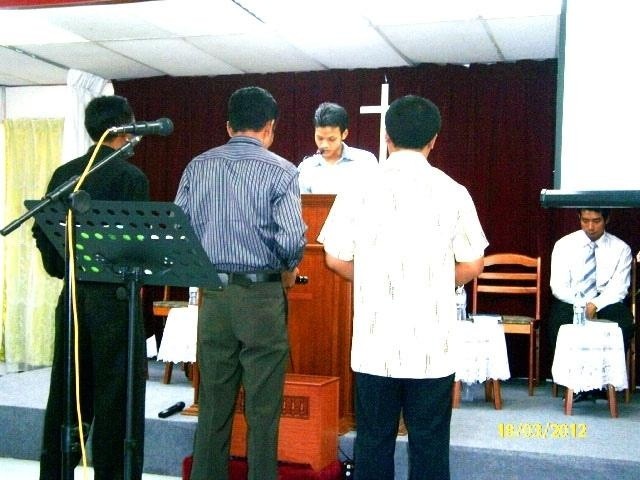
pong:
[295,275,308,283]
[159,401,185,418]
[109,118,174,137]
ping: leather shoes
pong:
[562,389,586,403]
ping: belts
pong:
[213,272,281,283]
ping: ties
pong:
[584,242,598,301]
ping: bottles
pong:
[573,283,586,327]
[454,283,466,321]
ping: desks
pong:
[182,193,355,436]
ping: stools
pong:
[452,315,511,411]
[553,321,627,416]
[156,307,199,384]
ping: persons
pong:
[167,88,308,478]
[293,102,380,195]
[314,94,489,479]
[33,95,150,479]
[542,208,633,410]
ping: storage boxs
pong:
[229,373,340,471]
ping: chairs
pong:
[472,254,542,398]
[626,258,636,404]
